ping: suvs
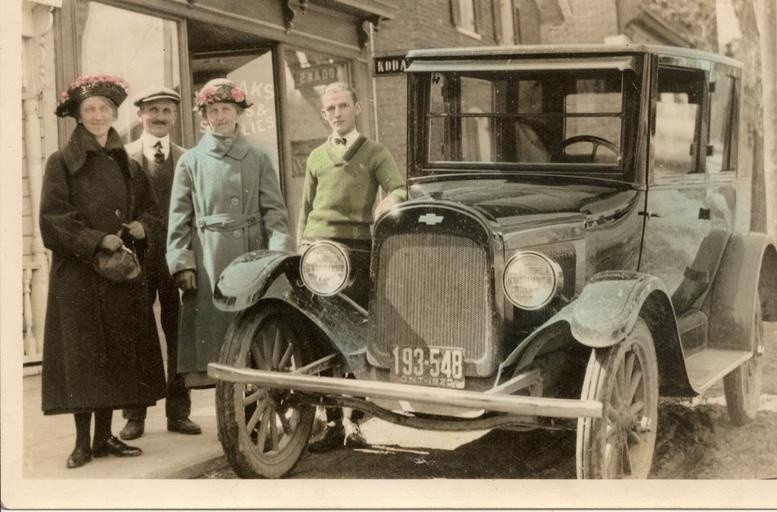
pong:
[203,41,777,480]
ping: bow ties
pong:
[335,138,346,146]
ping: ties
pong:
[152,142,164,182]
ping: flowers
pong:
[192,84,246,105]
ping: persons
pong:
[166,76,298,445]
[38,92,166,469]
[119,85,204,440]
[296,81,409,454]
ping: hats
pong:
[192,78,254,112]
[55,76,128,117]
[134,86,182,106]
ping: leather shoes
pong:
[120,421,144,439]
[67,433,142,468]
[167,418,201,434]
[307,423,366,450]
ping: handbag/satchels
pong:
[91,227,143,285]
